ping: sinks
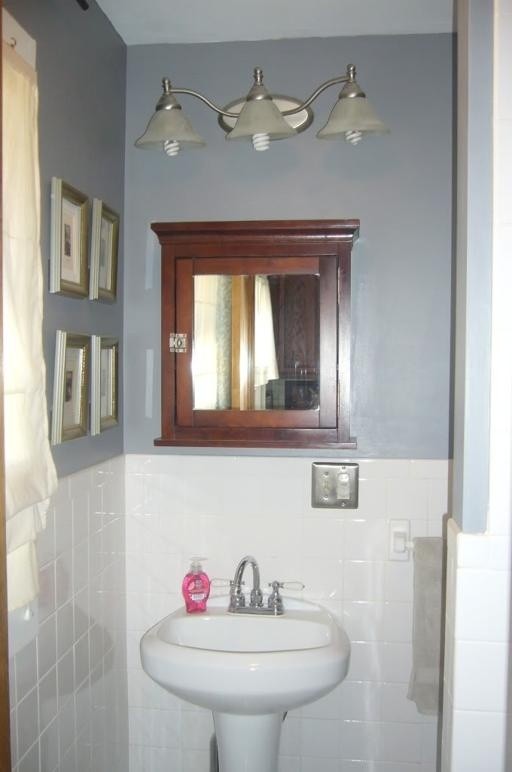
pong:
[140,607,350,716]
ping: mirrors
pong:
[175,254,337,430]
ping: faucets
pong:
[229,556,263,607]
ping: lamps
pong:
[135,64,389,157]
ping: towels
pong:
[404,535,446,717]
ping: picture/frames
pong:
[45,176,93,299]
[90,334,121,437]
[48,330,91,446]
[85,197,121,303]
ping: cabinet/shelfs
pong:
[275,275,320,378]
